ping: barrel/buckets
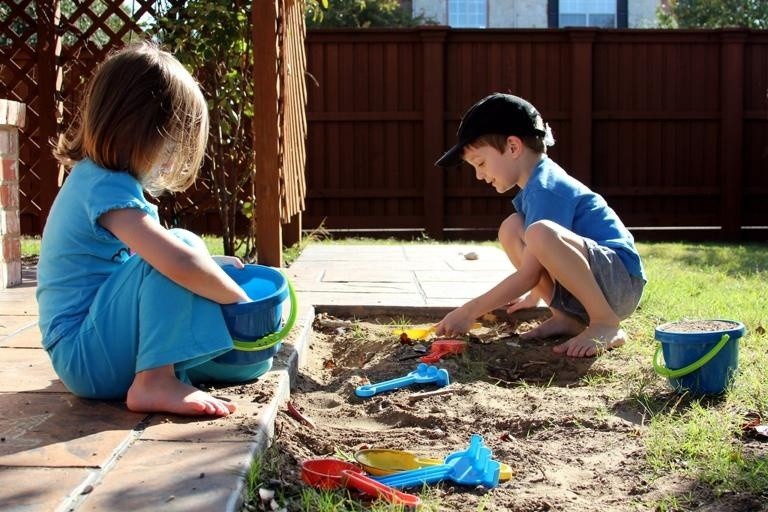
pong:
[186,263,296,384]
[652,319,744,395]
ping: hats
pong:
[434,92,547,169]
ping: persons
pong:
[433,92,647,357]
[35,43,254,416]
